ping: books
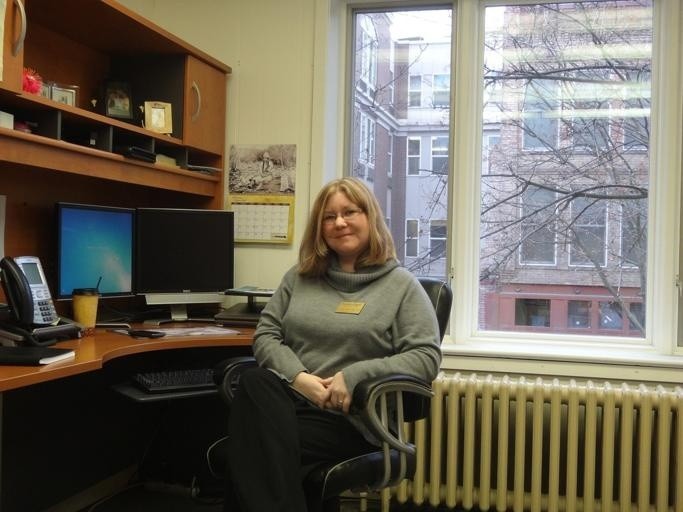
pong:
[0,346,75,368]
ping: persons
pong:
[220,176,444,512]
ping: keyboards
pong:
[131,368,240,394]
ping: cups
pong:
[71,287,99,337]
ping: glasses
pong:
[322,209,363,225]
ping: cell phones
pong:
[128,331,165,338]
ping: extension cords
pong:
[144,481,200,498]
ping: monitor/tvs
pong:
[136,208,234,325]
[54,202,136,329]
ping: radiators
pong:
[380,371,683,511]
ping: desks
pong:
[0,322,257,392]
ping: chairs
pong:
[205,275,451,511]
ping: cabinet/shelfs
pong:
[0,1,233,197]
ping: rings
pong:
[337,401,342,405]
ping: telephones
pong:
[0,255,58,328]
